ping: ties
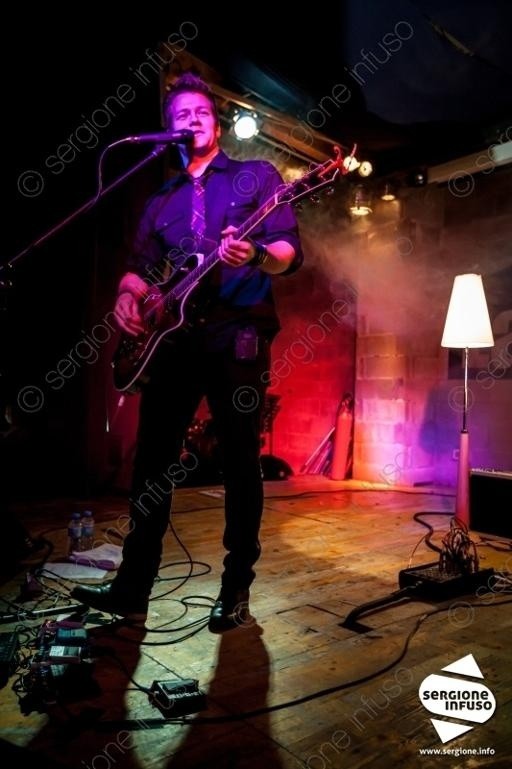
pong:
[190,171,217,252]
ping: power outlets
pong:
[452,448,460,460]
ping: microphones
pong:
[126,129,195,144]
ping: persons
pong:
[72,73,304,630]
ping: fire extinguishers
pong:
[329,391,354,480]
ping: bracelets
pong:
[247,240,268,270]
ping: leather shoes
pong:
[70,582,148,621]
[209,588,249,634]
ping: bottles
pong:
[66,512,84,554]
[81,509,96,551]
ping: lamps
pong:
[231,106,259,141]
[437,274,494,533]
[345,181,375,214]
[379,181,398,195]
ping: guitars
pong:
[112,144,358,396]
